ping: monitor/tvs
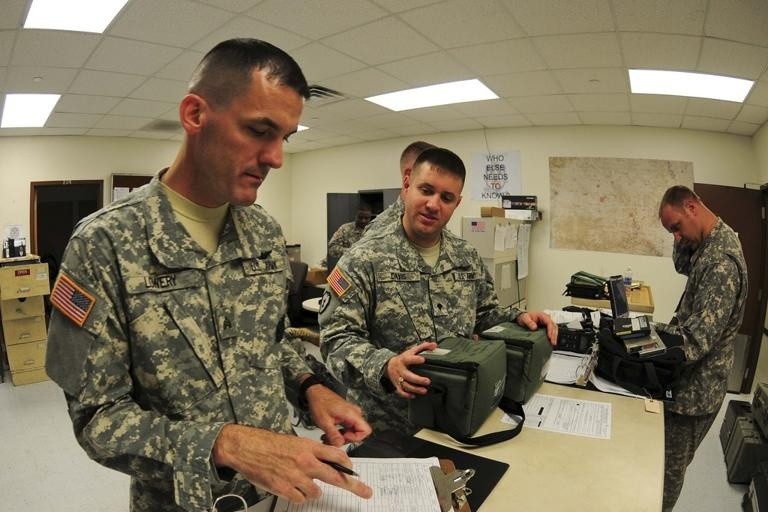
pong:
[607,274,629,319]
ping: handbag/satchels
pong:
[409,335,507,440]
[596,327,688,403]
[479,321,555,405]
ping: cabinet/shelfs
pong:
[1,254,53,387]
[461,216,529,316]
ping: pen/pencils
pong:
[323,461,359,478]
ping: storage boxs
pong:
[478,196,539,220]
[305,266,327,285]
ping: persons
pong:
[360,140,437,237]
[319,147,561,440]
[44,35,375,511]
[648,184,747,512]
[327,202,380,260]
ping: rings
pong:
[397,376,404,390]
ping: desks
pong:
[569,284,655,313]
[243,324,665,512]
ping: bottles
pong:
[623,266,633,297]
[18,237,26,256]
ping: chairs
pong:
[291,260,307,295]
